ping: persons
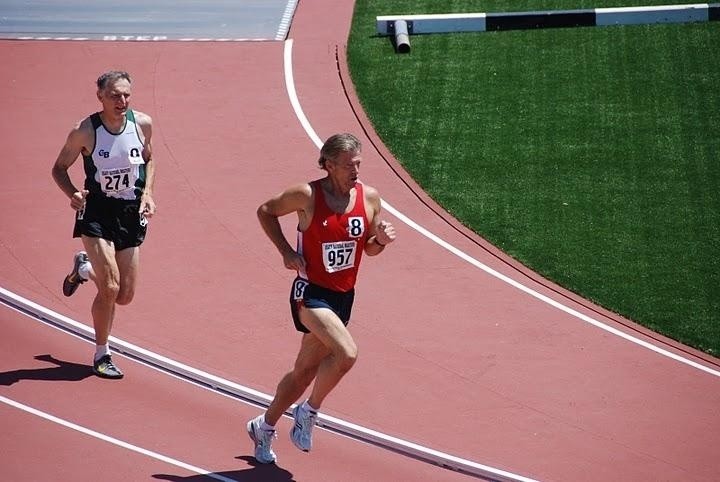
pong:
[246,132,396,465]
[51,72,156,380]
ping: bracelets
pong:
[375,237,385,246]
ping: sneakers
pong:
[245,416,277,463]
[289,401,318,452]
[93,353,124,379]
[62,250,88,297]
